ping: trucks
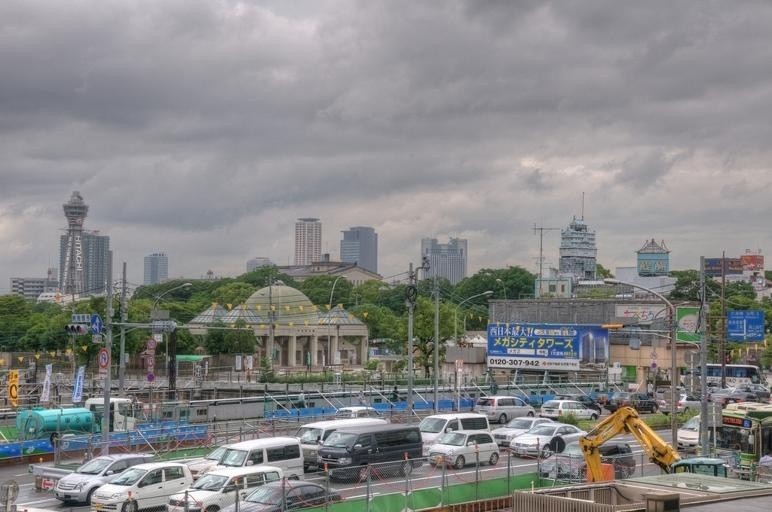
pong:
[15,394,141,434]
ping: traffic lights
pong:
[65,323,89,335]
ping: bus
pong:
[696,362,764,384]
[719,408,771,459]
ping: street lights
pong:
[149,283,192,408]
[328,276,348,367]
[454,290,494,360]
[604,278,678,450]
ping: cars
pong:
[53,436,344,511]
[420,392,702,468]
[708,381,770,403]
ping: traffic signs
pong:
[728,310,765,343]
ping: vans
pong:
[297,415,423,483]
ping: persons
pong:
[245,368,250,383]
[390,386,399,399]
[298,390,306,408]
[648,380,653,397]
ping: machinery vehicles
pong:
[578,406,728,484]
